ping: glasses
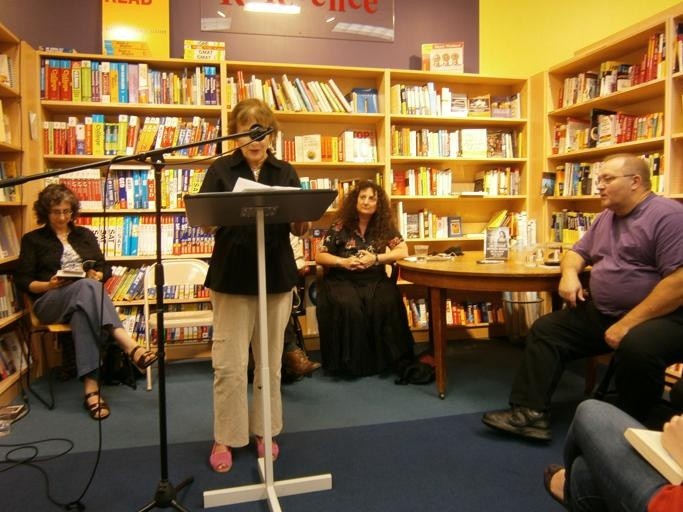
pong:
[595,175,637,184]
[48,209,74,216]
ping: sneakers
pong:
[482,407,553,439]
[544,463,568,508]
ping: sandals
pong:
[84,392,110,420]
[210,444,232,473]
[255,436,279,461]
[130,346,158,369]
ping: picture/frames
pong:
[420,41,465,73]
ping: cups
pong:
[523,248,542,267]
[413,244,429,262]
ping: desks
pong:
[392,248,589,400]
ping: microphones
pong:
[248,124,266,141]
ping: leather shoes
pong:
[285,349,322,375]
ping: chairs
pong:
[25,277,73,410]
[142,258,213,391]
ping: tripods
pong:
[133,167,196,511]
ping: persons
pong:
[546,400,682,511]
[15,183,158,419]
[478,154,682,441]
[315,178,416,381]
[246,231,322,380]
[197,97,310,473]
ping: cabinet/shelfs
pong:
[542,2,682,262]
[0,21,37,418]
[389,70,530,344]
[226,61,389,338]
[38,55,225,361]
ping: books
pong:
[42,115,222,157]
[105,265,214,346]
[301,227,330,261]
[557,22,682,108]
[548,210,601,246]
[274,127,383,209]
[40,58,222,105]
[624,426,682,485]
[1,55,35,383]
[391,167,525,239]
[44,164,209,209]
[540,108,664,196]
[226,70,379,113]
[391,82,526,158]
[401,290,539,326]
[74,215,216,257]
